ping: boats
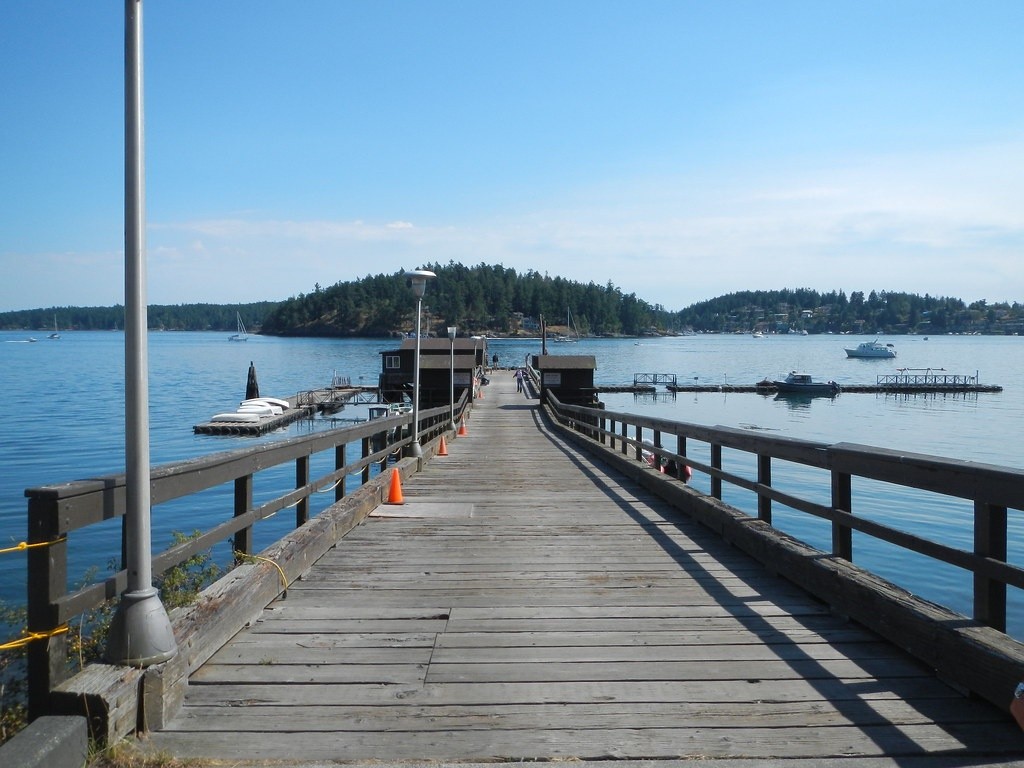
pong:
[751,331,766,338]
[26,337,38,342]
[843,337,899,360]
[236,398,290,418]
[772,371,843,396]
[628,436,691,481]
[211,414,261,424]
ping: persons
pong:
[525,353,531,368]
[512,366,528,393]
[492,352,499,371]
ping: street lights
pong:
[471,336,482,378]
[401,269,437,459]
[446,326,458,430]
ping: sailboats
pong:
[47,313,62,339]
[227,311,250,341]
[554,306,582,343]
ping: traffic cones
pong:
[437,435,449,456]
[386,467,405,506]
[457,415,468,436]
